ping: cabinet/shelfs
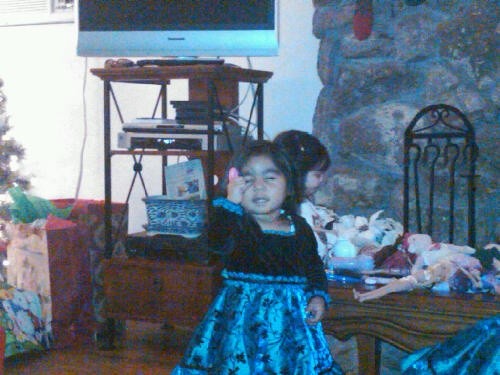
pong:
[89,60,275,354]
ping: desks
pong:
[320,280,500,375]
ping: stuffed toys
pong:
[314,206,500,303]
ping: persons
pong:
[272,129,332,269]
[170,140,346,375]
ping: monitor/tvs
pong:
[76,0,280,55]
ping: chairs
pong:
[402,102,482,253]
